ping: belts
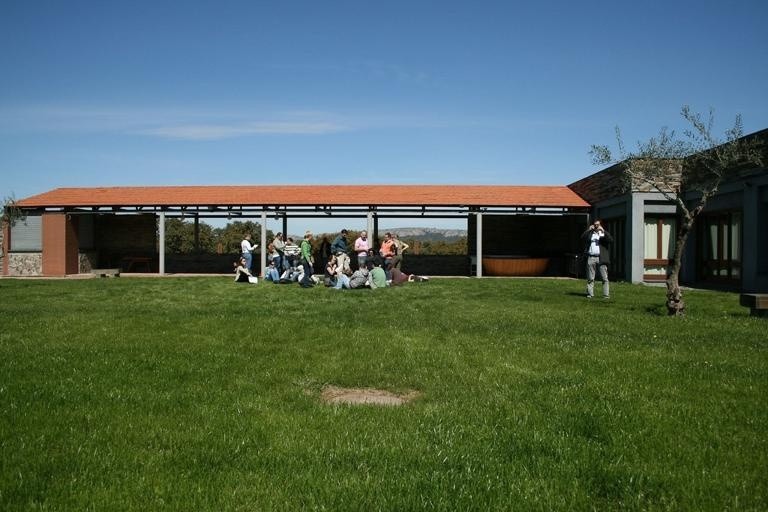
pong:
[590,255,600,257]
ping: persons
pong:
[580,217,614,299]
[234,227,411,289]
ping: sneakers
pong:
[587,295,609,299]
